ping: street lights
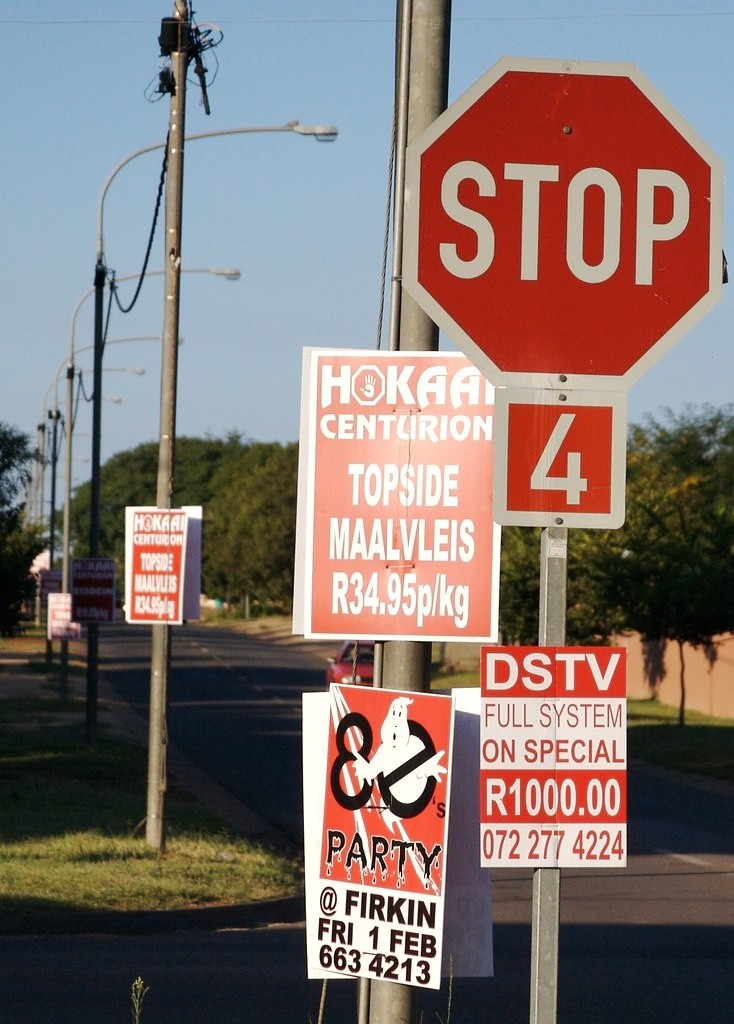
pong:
[33,332,182,675]
[58,266,242,709]
[81,121,340,747]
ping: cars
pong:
[326,639,375,690]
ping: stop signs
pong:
[400,49,723,391]
